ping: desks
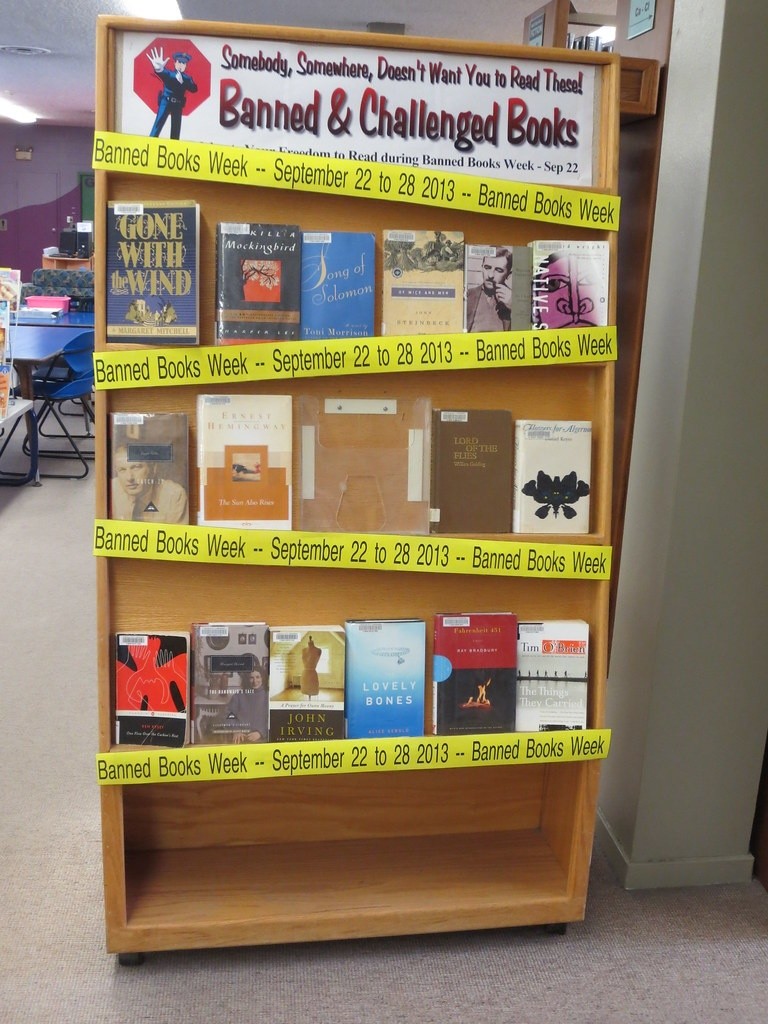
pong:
[0,326,94,449]
[0,400,39,487]
[0,312,94,326]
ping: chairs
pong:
[0,305,96,479]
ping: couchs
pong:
[21,269,94,312]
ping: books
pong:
[269,624,346,743]
[345,617,424,739]
[191,622,270,744]
[109,411,189,525]
[431,612,518,736]
[515,619,589,731]
[107,199,609,348]
[567,32,614,52]
[512,418,591,535]
[0,269,21,419]
[196,394,292,530]
[114,630,190,748]
[431,409,514,533]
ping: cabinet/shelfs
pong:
[42,254,94,269]
[94,12,622,967]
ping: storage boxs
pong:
[24,296,71,314]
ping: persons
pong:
[468,245,513,332]
[111,446,188,525]
[220,668,268,744]
[463,678,491,706]
